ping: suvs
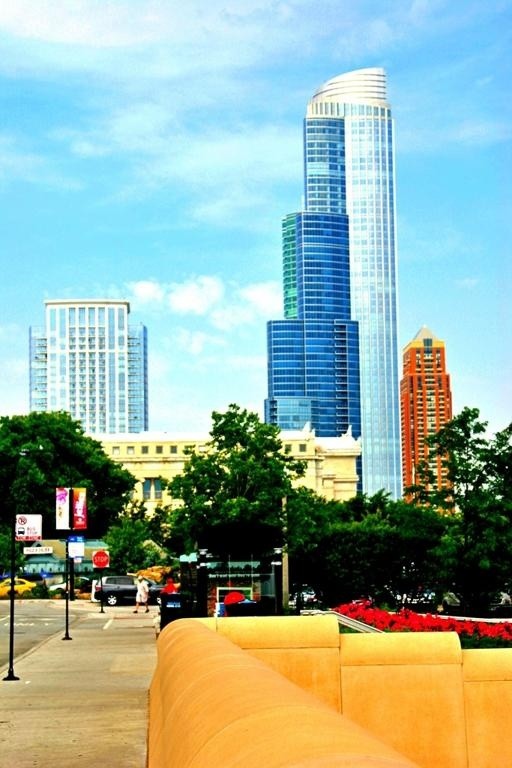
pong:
[94,574,166,605]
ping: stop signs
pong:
[92,550,109,568]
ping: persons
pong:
[132,575,150,613]
[159,577,179,593]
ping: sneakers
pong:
[133,609,149,613]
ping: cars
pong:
[0,577,36,597]
[48,576,91,596]
